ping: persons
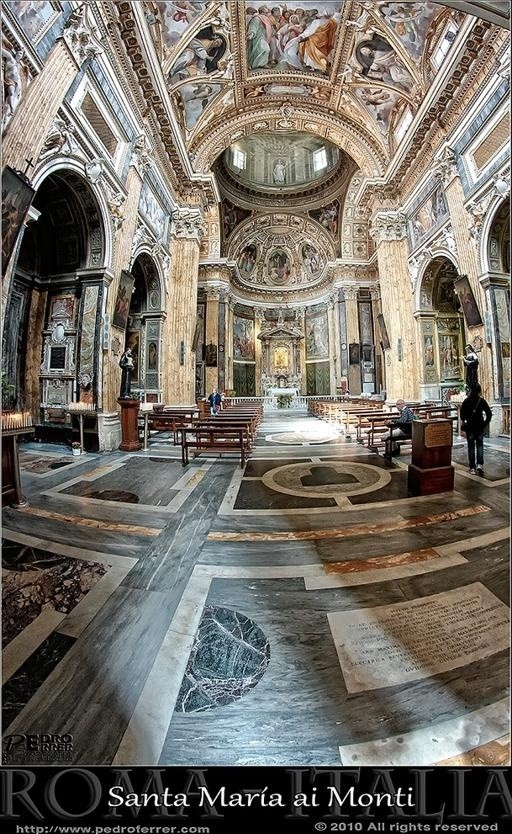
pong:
[274,160,286,182]
[168,38,223,78]
[379,2,434,42]
[384,400,415,456]
[460,383,492,475]
[38,124,75,160]
[360,46,413,90]
[119,348,135,399]
[2,49,24,115]
[361,88,391,110]
[461,345,480,390]
[245,4,337,74]
[208,388,221,415]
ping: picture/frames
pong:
[349,340,362,366]
[204,341,218,367]
[376,312,393,351]
[191,314,205,352]
[454,274,484,327]
[437,331,463,380]
[111,268,136,332]
[48,292,79,328]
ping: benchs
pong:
[136,400,266,467]
[308,394,459,463]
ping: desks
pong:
[266,386,297,405]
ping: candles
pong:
[67,401,96,410]
[0,409,32,431]
